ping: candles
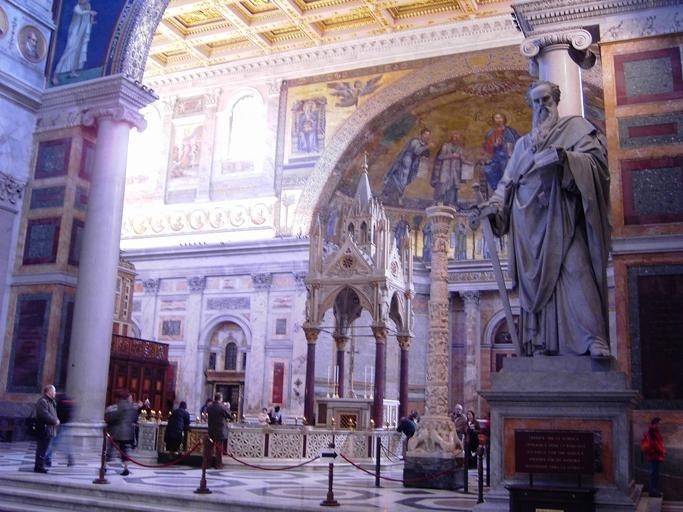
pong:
[327,366,340,385]
[364,366,373,382]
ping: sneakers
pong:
[33,465,50,474]
[119,469,129,476]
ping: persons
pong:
[162,399,190,453]
[105,392,150,474]
[396,410,419,460]
[451,404,479,469]
[259,406,282,425]
[52,0,97,86]
[296,102,317,152]
[35,386,74,472]
[308,413,315,425]
[477,81,615,361]
[646,417,668,497]
[202,392,234,442]
[22,29,39,59]
[380,114,522,263]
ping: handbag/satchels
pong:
[24,416,46,436]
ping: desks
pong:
[504,484,599,512]
